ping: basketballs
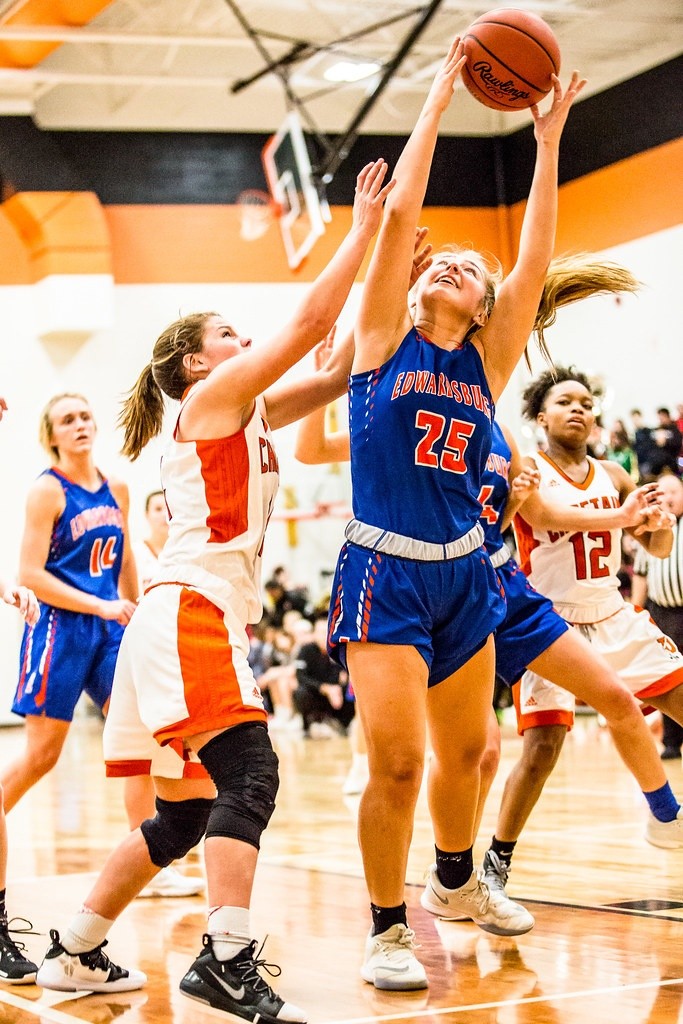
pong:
[458,6,562,112]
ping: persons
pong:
[248,406,683,796]
[630,474,683,758]
[326,33,589,991]
[0,395,205,985]
[34,156,434,1024]
[293,319,683,884]
[478,364,683,921]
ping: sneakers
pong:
[37,929,147,992]
[643,806,683,849]
[480,849,508,899]
[0,911,38,985]
[344,752,369,793]
[363,922,428,990]
[421,863,535,936]
[180,933,310,1024]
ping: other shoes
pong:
[661,745,682,760]
[309,714,347,739]
